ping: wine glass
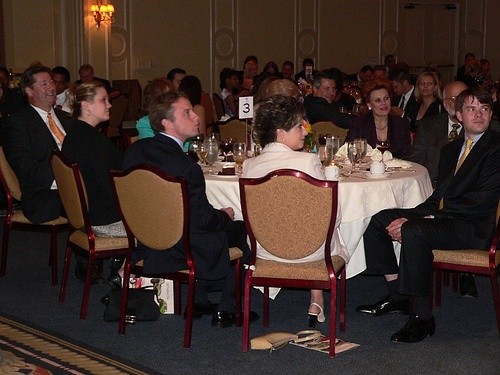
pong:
[188,133,234,167]
[252,126,263,157]
[347,142,361,175]
[354,138,367,171]
[203,139,218,175]
[233,143,246,176]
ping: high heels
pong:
[307,303,325,328]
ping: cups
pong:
[338,102,367,118]
[371,162,389,174]
[318,132,340,166]
[325,166,339,179]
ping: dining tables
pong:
[140,142,434,316]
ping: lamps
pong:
[91,4,114,28]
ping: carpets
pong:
[0,315,152,375]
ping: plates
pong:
[365,173,391,179]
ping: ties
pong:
[307,74,310,79]
[439,140,473,209]
[399,96,405,110]
[448,124,461,142]
[359,81,363,87]
[47,112,65,143]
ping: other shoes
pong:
[76,267,106,286]
[104,299,159,323]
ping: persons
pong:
[122,91,258,327]
[0,66,71,224]
[60,79,137,288]
[358,86,500,344]
[412,80,478,298]
[457,53,493,90]
[135,78,192,153]
[241,95,342,326]
[167,68,206,135]
[352,54,446,132]
[218,56,320,117]
[51,66,72,113]
[304,71,357,129]
[343,83,410,158]
[73,64,111,89]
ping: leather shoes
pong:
[185,302,215,319]
[211,311,260,328]
[355,295,410,316]
[390,313,435,343]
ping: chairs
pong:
[0,71,500,358]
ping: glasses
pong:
[444,96,458,103]
